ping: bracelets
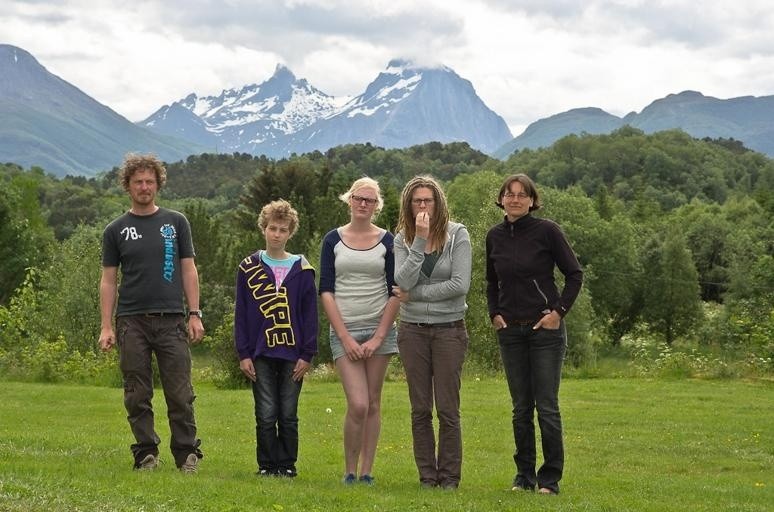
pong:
[188,310,203,319]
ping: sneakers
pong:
[257,468,278,477]
[175,449,197,474]
[360,476,374,485]
[280,467,297,477]
[134,450,159,470]
[345,473,356,483]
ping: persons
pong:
[391,176,476,492]
[482,171,585,497]
[231,198,319,479]
[94,147,208,477]
[317,175,399,487]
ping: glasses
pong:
[410,199,436,205]
[504,192,529,199]
[352,195,377,206]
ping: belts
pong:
[133,313,185,317]
[503,318,540,325]
[401,320,465,328]
